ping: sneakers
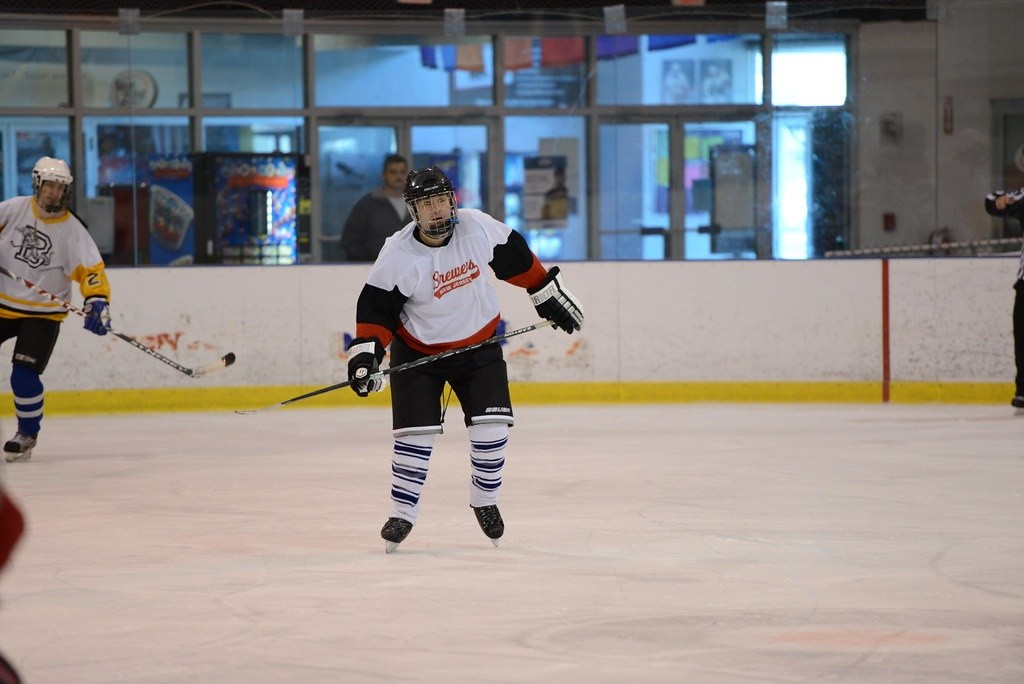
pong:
[470,504,504,547]
[3,432,36,463]
[1011,395,1024,416]
[380,517,413,554]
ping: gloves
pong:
[526,265,584,335]
[82,294,111,336]
[347,335,386,397]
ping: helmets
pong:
[402,165,458,240]
[32,156,73,212]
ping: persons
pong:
[0,156,114,462]
[342,153,414,260]
[985,147,1024,408]
[348,165,584,553]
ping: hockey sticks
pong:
[234,318,551,417]
[0,265,235,379]
